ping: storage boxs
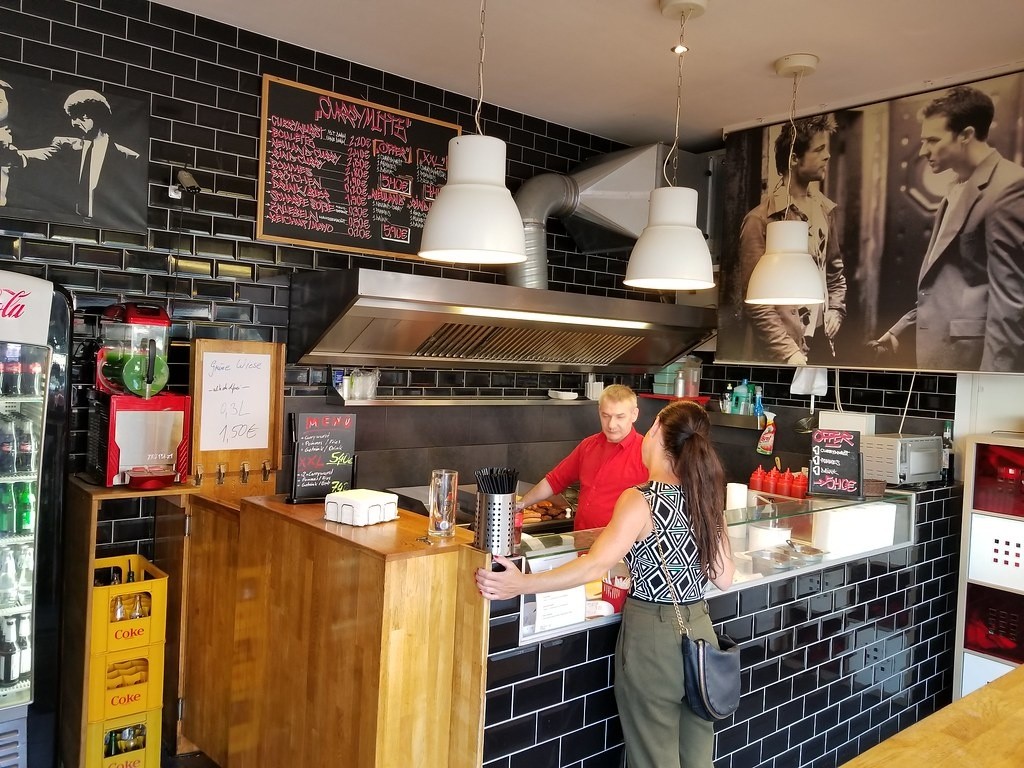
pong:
[652,360,685,396]
[84,555,170,654]
[80,708,162,768]
[324,489,400,526]
[85,644,165,724]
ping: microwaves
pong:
[858,432,943,485]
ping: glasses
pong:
[789,203,809,222]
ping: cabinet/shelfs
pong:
[952,432,1024,700]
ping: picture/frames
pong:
[255,74,463,265]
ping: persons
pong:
[867,86,1024,374]
[519,384,651,560]
[474,402,735,768]
[736,114,847,368]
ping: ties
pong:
[78,139,94,216]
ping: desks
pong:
[839,657,1024,768]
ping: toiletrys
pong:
[717,378,764,417]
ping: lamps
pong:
[419,0,530,264]
[746,53,824,307]
[622,1,715,292]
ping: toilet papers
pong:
[726,481,747,540]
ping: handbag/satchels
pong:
[680,633,742,720]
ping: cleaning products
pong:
[756,411,777,455]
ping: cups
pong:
[429,469,458,540]
[514,502,524,549]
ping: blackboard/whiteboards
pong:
[292,411,359,504]
[808,428,861,498]
[255,74,463,266]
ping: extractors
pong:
[289,267,716,376]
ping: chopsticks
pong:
[476,467,520,494]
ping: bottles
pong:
[0,481,36,537]
[0,343,42,396]
[720,379,767,415]
[112,595,129,621]
[0,613,33,687]
[0,413,36,477]
[131,595,145,618]
[106,725,144,757]
[342,377,351,400]
[940,420,954,486]
[749,465,808,498]
[0,544,34,607]
[673,370,687,397]
[94,570,134,586]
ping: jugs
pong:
[685,367,701,396]
